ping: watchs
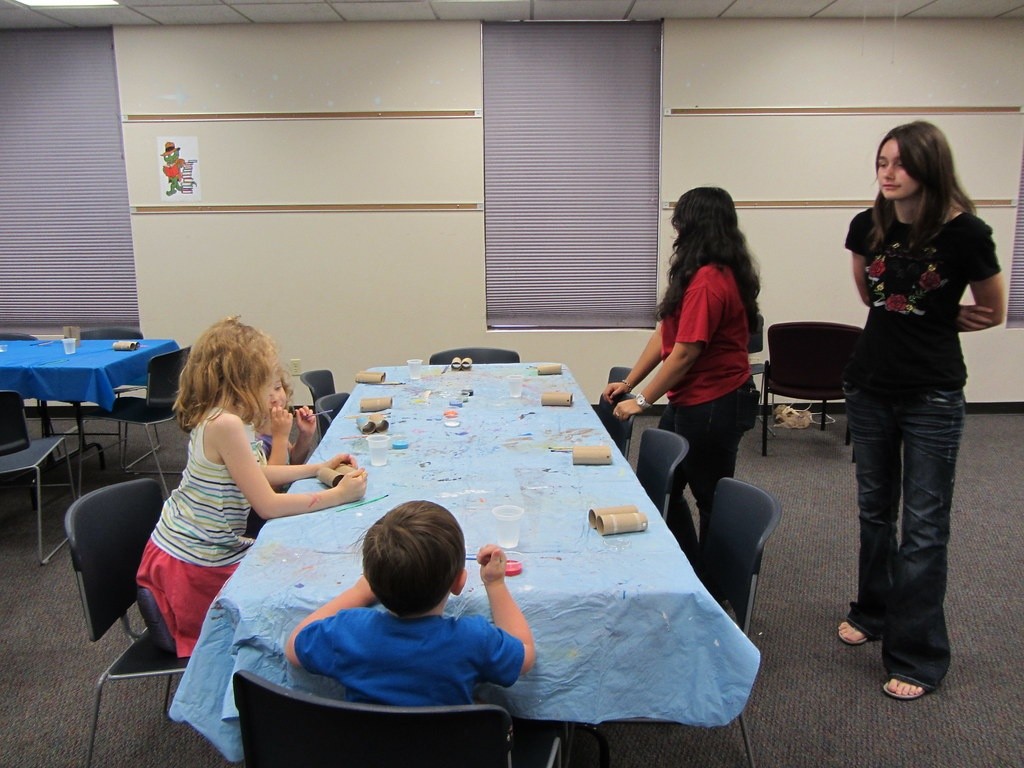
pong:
[635,393,652,411]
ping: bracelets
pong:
[621,380,632,393]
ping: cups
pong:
[492,504,524,549]
[61,337,76,354]
[509,375,523,398]
[407,359,423,380]
[366,434,390,467]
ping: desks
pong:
[0,340,180,511]
[168,362,763,768]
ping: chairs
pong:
[0,322,864,768]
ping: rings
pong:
[615,412,620,417]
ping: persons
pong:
[136,312,366,661]
[604,187,763,610]
[837,123,1005,701]
[286,500,538,707]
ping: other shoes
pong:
[883,679,925,699]
[839,621,868,645]
